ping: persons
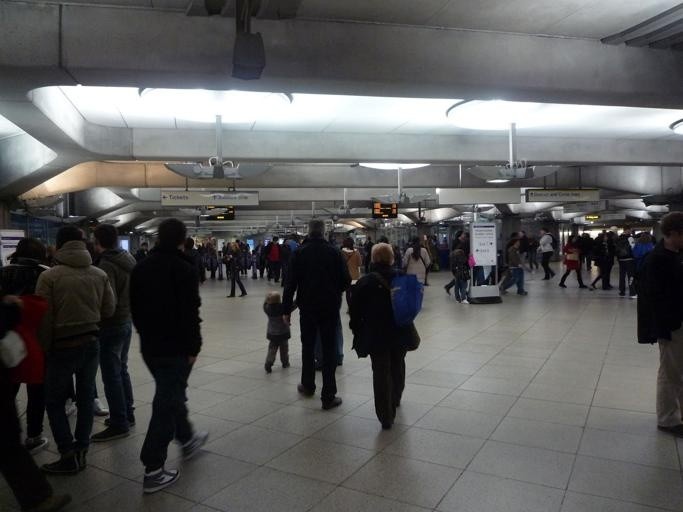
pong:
[91,224,137,442]
[445,231,468,295]
[129,219,209,494]
[340,237,362,315]
[636,211,683,439]
[632,232,655,273]
[1,295,72,512]
[559,234,588,288]
[500,239,528,295]
[347,243,424,430]
[616,225,637,299]
[449,244,470,304]
[222,242,247,298]
[183,234,266,281]
[289,233,305,250]
[35,226,116,478]
[280,238,292,287]
[598,233,611,290]
[135,242,149,260]
[499,232,521,294]
[528,232,540,270]
[421,234,439,271]
[519,230,535,275]
[282,218,352,411]
[355,236,407,273]
[592,232,616,289]
[263,292,298,374]
[536,227,555,280]
[0,238,52,456]
[402,237,430,286]
[266,236,282,282]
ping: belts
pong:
[53,335,101,352]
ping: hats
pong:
[266,292,281,304]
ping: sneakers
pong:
[517,289,527,296]
[74,443,88,471]
[322,397,342,410]
[381,421,391,429]
[298,382,315,397]
[62,399,75,418]
[144,470,181,497]
[24,494,71,512]
[498,286,508,293]
[456,301,460,304]
[27,437,49,453]
[42,458,80,477]
[92,424,131,442]
[93,399,110,417]
[394,402,402,405]
[282,362,289,366]
[264,363,272,374]
[178,431,209,459]
[104,416,137,428]
[461,299,470,304]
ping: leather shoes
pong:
[609,283,614,289]
[239,293,248,298]
[541,276,550,281]
[656,421,683,439]
[591,283,596,288]
[551,273,555,277]
[227,294,236,298]
[559,283,568,289]
[619,291,624,295]
[579,285,586,288]
[629,292,636,296]
[602,287,608,290]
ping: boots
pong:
[445,279,452,296]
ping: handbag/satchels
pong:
[463,267,472,280]
[372,269,421,350]
[412,247,427,267]
[0,329,29,367]
[390,271,423,325]
[344,250,355,267]
